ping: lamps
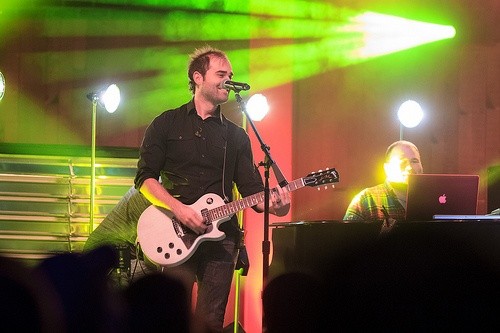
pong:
[397,99,424,140]
[86,83,121,234]
[236,94,269,131]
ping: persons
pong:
[133,44,291,333]
[83,174,163,290]
[342,139,425,221]
[0,242,341,332]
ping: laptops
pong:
[407,174,479,222]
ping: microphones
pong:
[221,80,250,90]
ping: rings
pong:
[277,199,281,203]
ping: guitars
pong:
[137,168,339,269]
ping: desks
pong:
[270,219,500,274]
